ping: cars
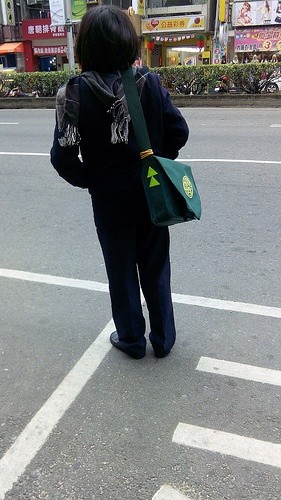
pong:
[256,76,281,93]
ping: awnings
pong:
[0,42,24,53]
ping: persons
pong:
[236,2,250,25]
[75,61,78,76]
[135,57,140,66]
[263,1,271,24]
[50,5,202,359]
[222,51,281,64]
[274,1,281,23]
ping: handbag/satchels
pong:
[142,155,202,226]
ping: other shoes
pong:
[110,331,146,359]
